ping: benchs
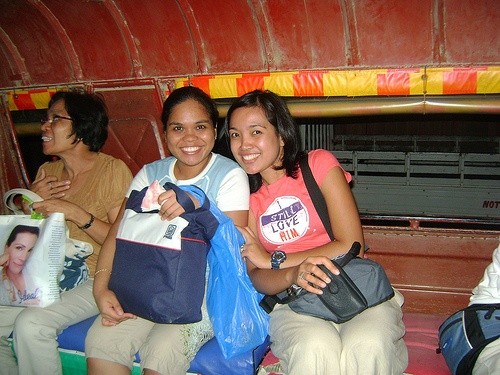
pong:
[0,226,500,375]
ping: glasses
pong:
[41,114,73,127]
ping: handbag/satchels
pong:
[0,187,94,308]
[437,302,500,375]
[286,251,394,324]
[107,182,219,324]
[177,185,269,359]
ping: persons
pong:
[85,85,249,375]
[0,91,133,375]
[0,225,40,304]
[468,243,500,375]
[227,90,409,375]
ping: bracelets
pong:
[79,213,94,230]
[95,269,111,277]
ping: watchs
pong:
[271,250,286,270]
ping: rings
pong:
[241,244,244,253]
